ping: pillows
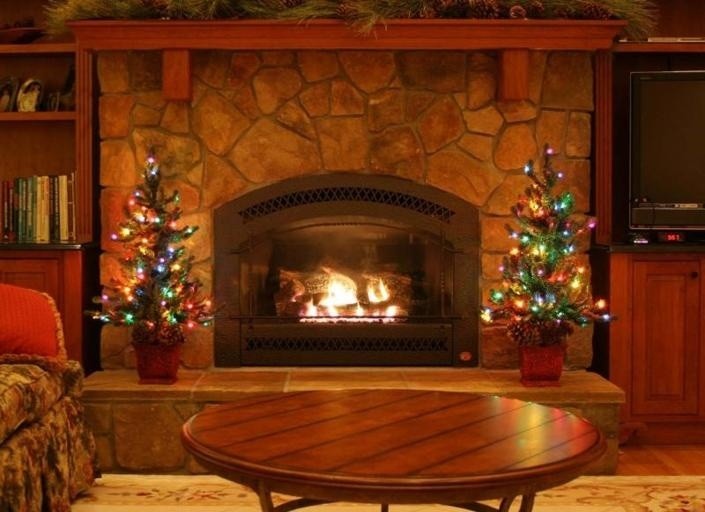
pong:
[0,283,71,375]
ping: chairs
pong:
[2,359,103,511]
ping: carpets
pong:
[70,470,705,512]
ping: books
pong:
[0,172,74,244]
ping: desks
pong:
[180,390,610,511]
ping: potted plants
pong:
[83,145,228,388]
[476,144,620,389]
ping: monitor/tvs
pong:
[629,71,705,234]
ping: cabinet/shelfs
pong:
[595,254,705,448]
[0,41,93,245]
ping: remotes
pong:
[632,236,648,244]
